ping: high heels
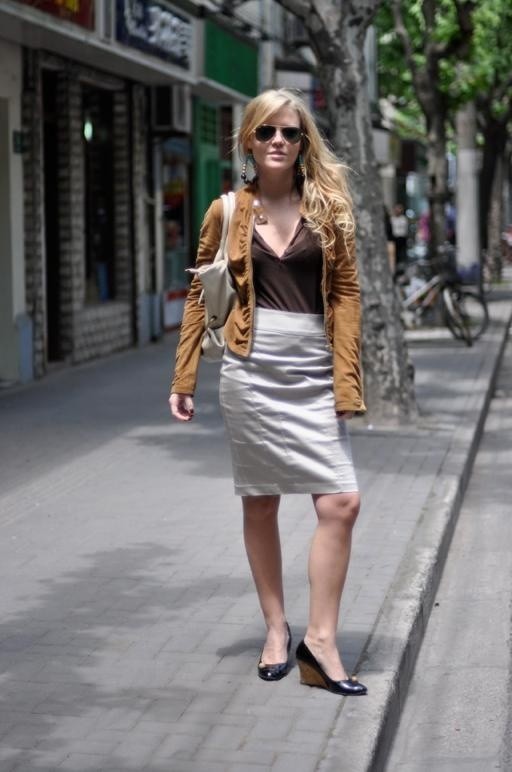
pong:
[259,622,292,682]
[297,640,367,696]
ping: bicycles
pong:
[394,253,489,347]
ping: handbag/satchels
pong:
[185,192,236,361]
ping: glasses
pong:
[256,124,306,144]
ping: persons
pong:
[166,86,370,697]
[384,195,457,287]
